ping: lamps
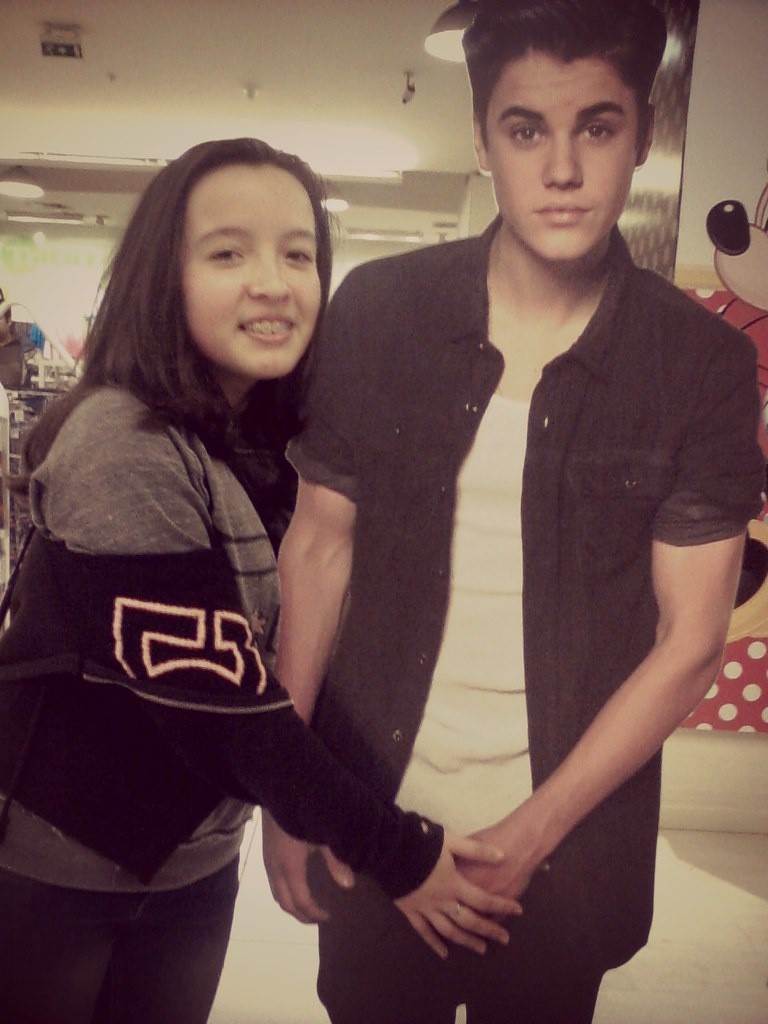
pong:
[0,164,44,199]
[424,0,476,62]
[321,179,349,212]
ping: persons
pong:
[261,0,762,1024]
[0,299,32,391]
[0,138,524,1024]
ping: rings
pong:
[451,902,462,921]
